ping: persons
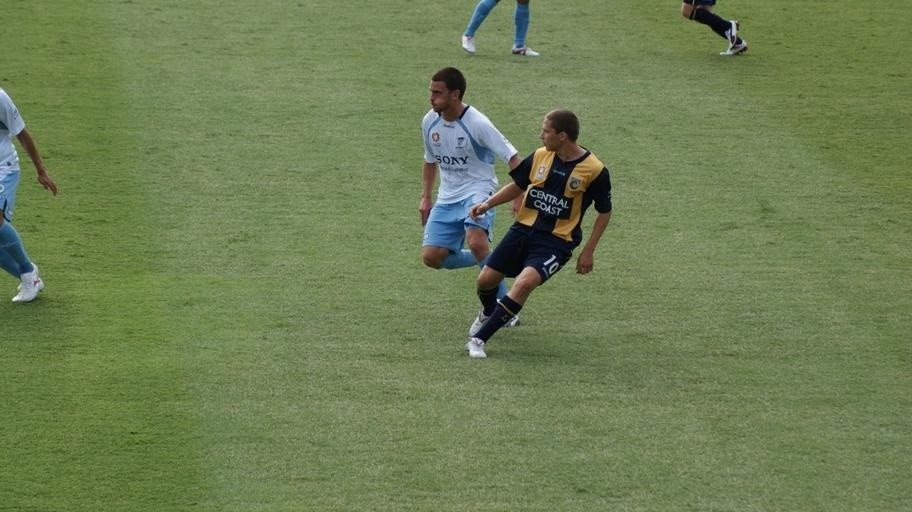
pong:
[465,109,612,358]
[0,87,57,302]
[462,0,540,56]
[419,67,525,327]
[681,0,747,55]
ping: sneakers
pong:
[512,46,539,56]
[465,310,519,359]
[720,20,748,56]
[12,262,45,302]
[462,34,476,54]
